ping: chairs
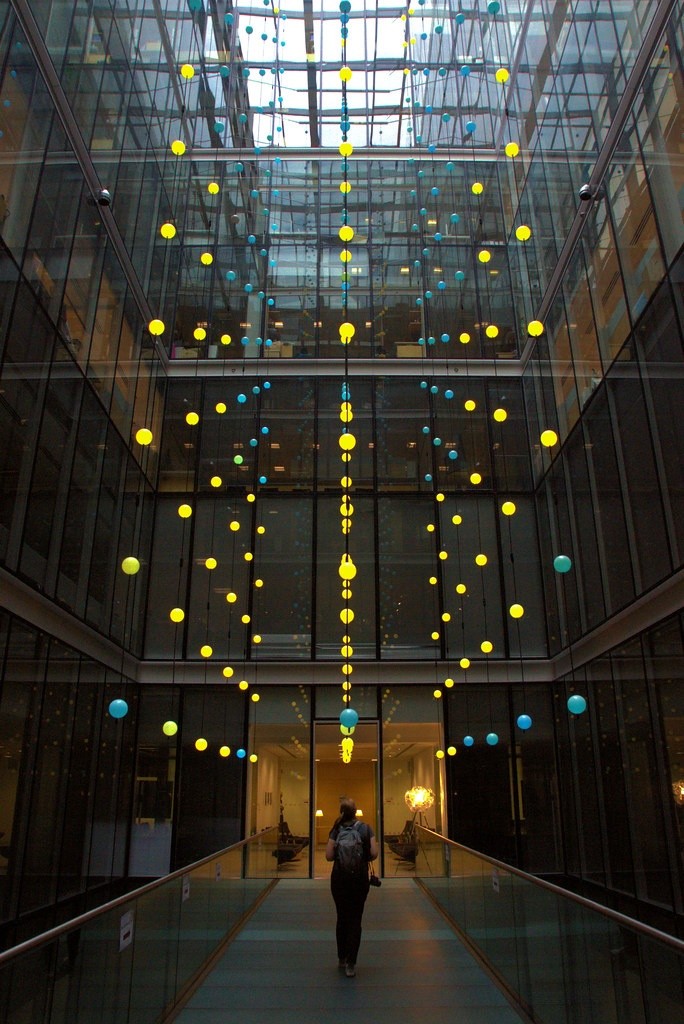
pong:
[384,820,429,862]
[263,822,309,858]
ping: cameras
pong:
[370,876,385,887]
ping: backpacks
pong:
[335,821,364,882]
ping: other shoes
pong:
[338,957,355,976]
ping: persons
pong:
[326,800,378,978]
